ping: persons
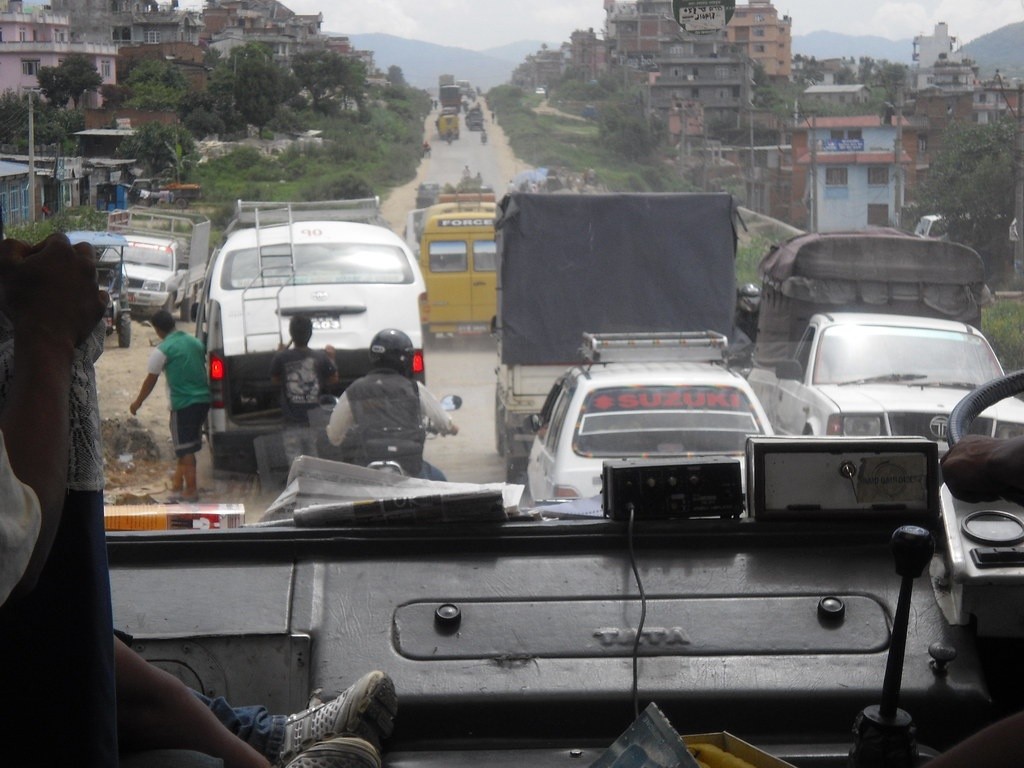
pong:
[112,631,397,768]
[429,85,494,145]
[0,234,107,608]
[937,431,1024,502]
[131,310,212,504]
[270,314,339,472]
[328,329,459,481]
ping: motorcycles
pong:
[318,393,461,478]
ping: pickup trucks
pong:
[745,229,1024,444]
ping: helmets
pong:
[370,329,415,370]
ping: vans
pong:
[131,178,152,190]
[406,182,501,344]
[203,221,431,468]
[522,360,775,504]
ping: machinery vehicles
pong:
[129,188,152,207]
[66,231,132,348]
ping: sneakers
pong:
[282,670,397,765]
[285,737,383,768]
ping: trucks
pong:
[491,186,738,487]
[437,74,483,142]
[101,209,211,323]
[916,214,951,243]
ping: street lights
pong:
[21,88,49,223]
